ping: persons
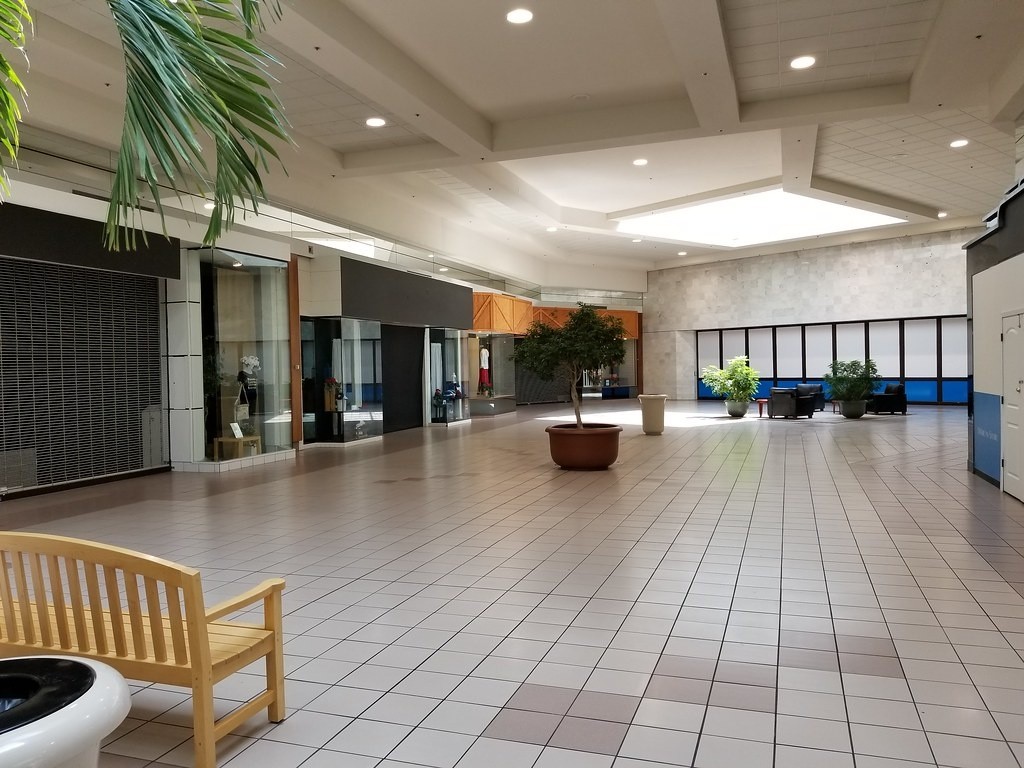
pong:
[237,364,258,415]
[451,372,457,383]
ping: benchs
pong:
[0,530,287,768]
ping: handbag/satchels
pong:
[234,404,250,420]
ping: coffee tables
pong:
[757,399,768,417]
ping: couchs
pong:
[767,387,815,419]
[865,384,908,415]
[796,384,825,411]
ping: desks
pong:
[832,400,868,414]
[214,436,262,463]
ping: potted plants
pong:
[504,301,633,470]
[701,355,762,417]
[823,359,883,419]
